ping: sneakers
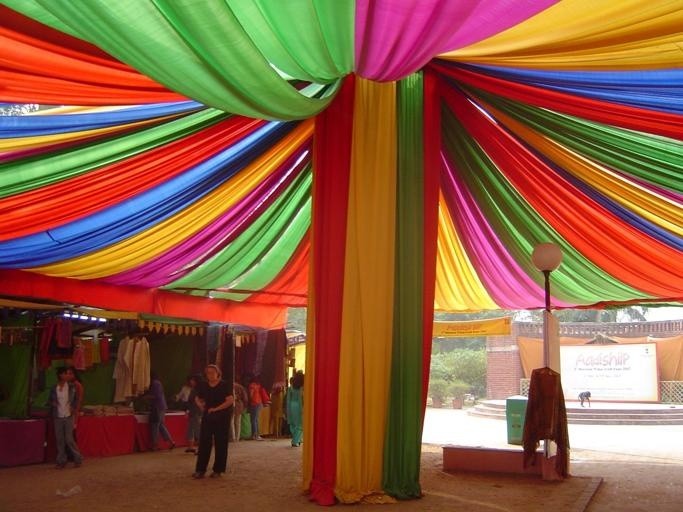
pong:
[55,461,80,469]
[190,471,205,478]
[209,471,220,478]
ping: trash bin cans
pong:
[505,394,528,445]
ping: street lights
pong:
[531,243,563,311]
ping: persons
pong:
[173,374,207,455]
[246,376,264,440]
[142,371,177,453]
[46,367,83,468]
[229,376,248,442]
[284,372,304,447]
[191,364,233,479]
